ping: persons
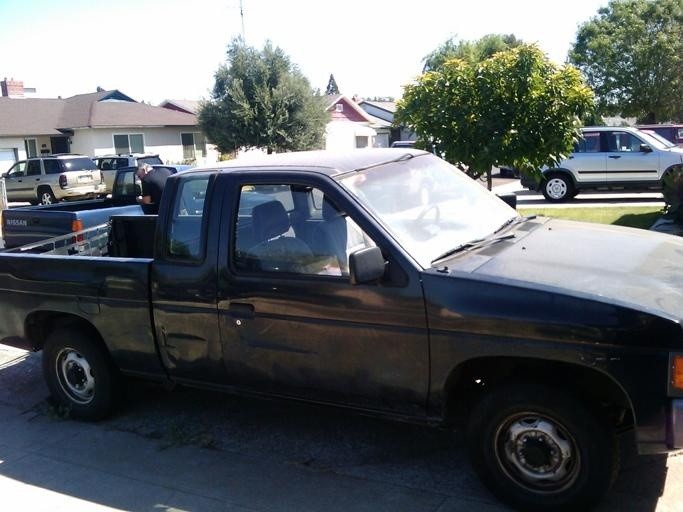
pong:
[133,163,174,217]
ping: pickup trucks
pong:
[0,147,683,512]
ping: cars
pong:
[0,152,193,248]
[390,123,683,203]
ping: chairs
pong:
[248,192,375,280]
